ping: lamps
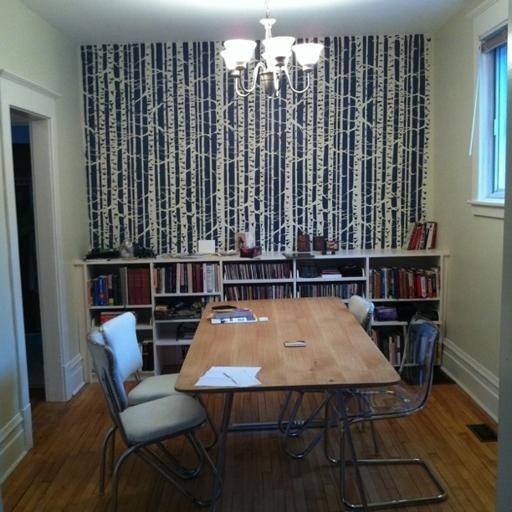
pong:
[217,3,327,103]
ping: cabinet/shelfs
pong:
[75,247,451,385]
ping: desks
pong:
[174,296,402,509]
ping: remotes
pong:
[285,340,306,347]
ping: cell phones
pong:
[211,304,236,311]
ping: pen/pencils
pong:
[224,373,238,384]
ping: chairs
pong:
[277,296,381,462]
[98,310,219,480]
[323,311,448,510]
[84,329,226,509]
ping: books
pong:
[222,232,293,301]
[87,266,154,372]
[369,222,442,365]
[296,234,366,300]
[153,240,257,360]
